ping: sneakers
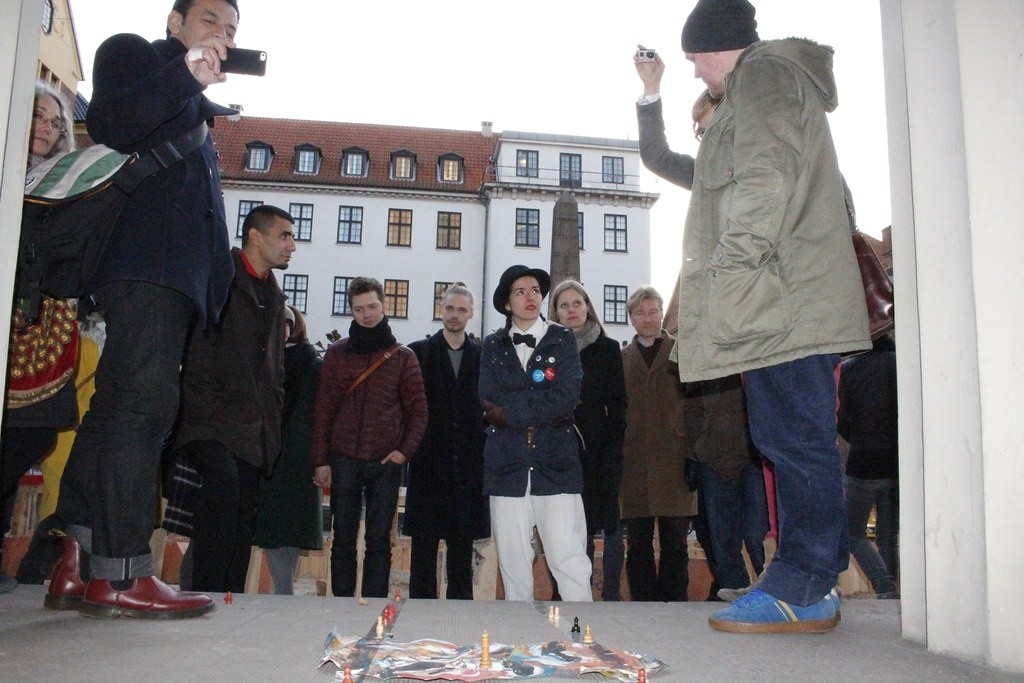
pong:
[708,578,841,634]
[717,576,766,602]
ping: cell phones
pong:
[216,48,266,77]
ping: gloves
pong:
[482,399,507,430]
[553,415,575,429]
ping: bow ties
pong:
[513,333,536,348]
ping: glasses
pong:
[33,110,67,135]
[693,121,707,143]
[630,310,661,318]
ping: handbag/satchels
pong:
[24,143,139,300]
[851,232,894,341]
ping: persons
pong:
[666,1,873,636]
[45,0,241,619]
[548,281,627,601]
[16,205,320,600]
[836,335,900,600]
[0,83,73,593]
[403,282,491,598]
[312,277,428,598]
[620,289,699,602]
[635,43,785,600]
[478,265,593,601]
[686,374,770,589]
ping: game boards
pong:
[315,619,668,682]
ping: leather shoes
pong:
[79,574,215,620]
[44,536,90,610]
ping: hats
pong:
[681,0,760,53]
[493,264,551,317]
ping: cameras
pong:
[638,49,656,63]
